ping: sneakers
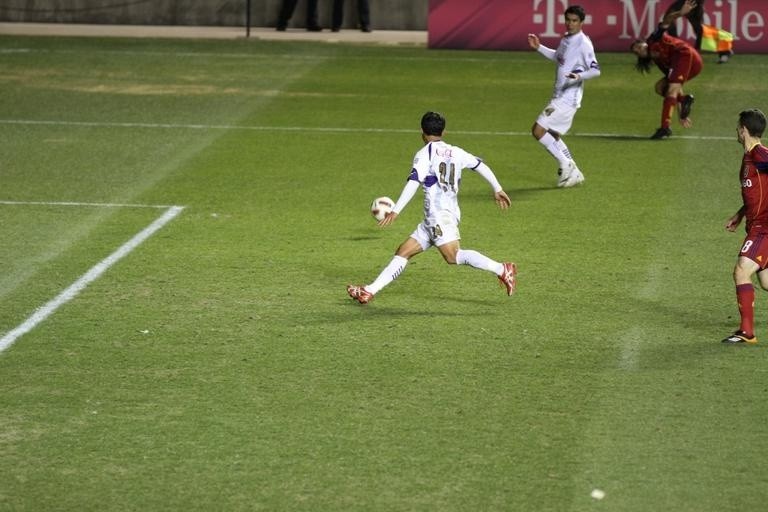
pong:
[721,330,757,343]
[681,94,694,119]
[347,285,374,304]
[650,127,672,139]
[718,55,729,65]
[498,262,517,296]
[558,160,575,188]
[565,172,584,188]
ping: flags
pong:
[702,22,733,51]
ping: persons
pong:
[527,4,603,190]
[276,1,327,33]
[630,0,705,139]
[720,108,768,344]
[658,0,734,65]
[344,110,519,304]
[332,2,373,34]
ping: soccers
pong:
[371,197,394,221]
